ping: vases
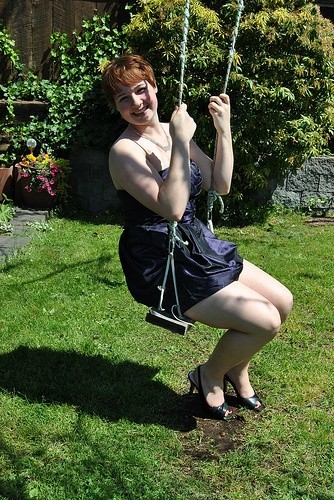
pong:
[13,162,52,209]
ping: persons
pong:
[101,54,294,423]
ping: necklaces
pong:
[128,123,171,152]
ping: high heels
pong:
[223,373,265,412]
[188,365,237,420]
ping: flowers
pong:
[16,151,67,195]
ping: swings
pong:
[145,0,243,335]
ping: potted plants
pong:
[0,153,16,202]
